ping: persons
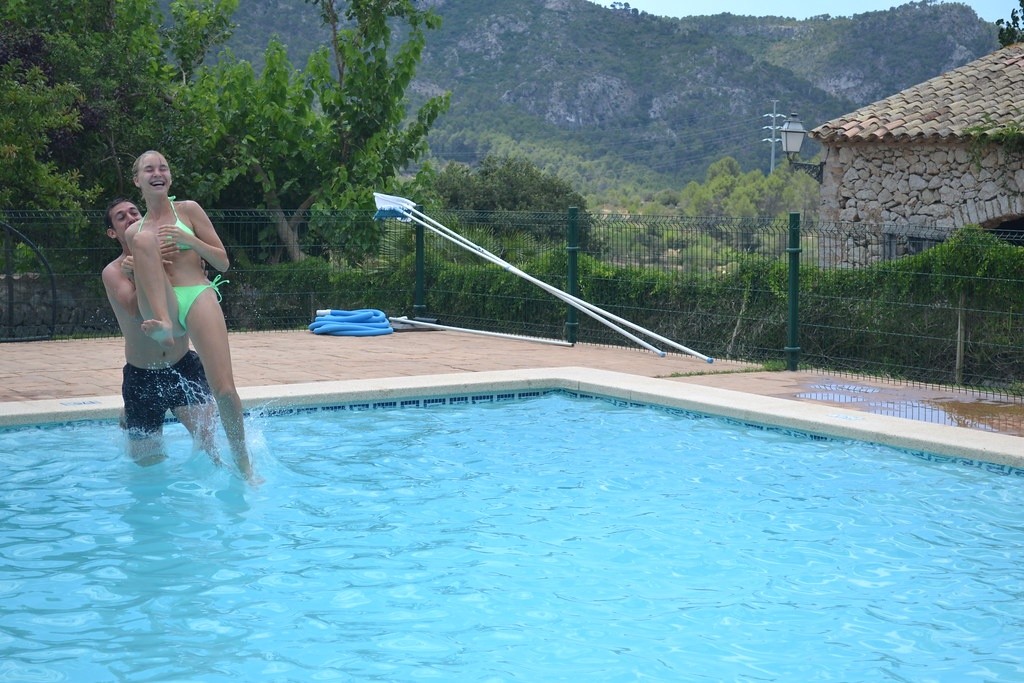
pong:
[121,149,244,445]
[101,199,216,435]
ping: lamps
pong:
[779,111,827,184]
